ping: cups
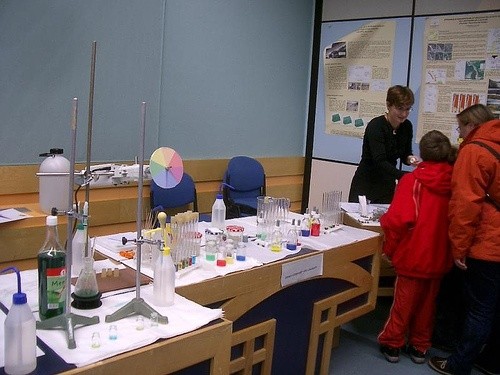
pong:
[268,199,290,233]
[256,196,272,229]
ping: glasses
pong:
[455,123,463,132]
[394,103,414,113]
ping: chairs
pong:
[149,173,211,228]
[223,155,267,220]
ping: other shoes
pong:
[378,343,400,362]
[428,356,472,375]
[400,344,426,364]
[473,359,498,375]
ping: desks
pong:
[0,258,234,375]
[89,207,382,375]
[338,205,393,299]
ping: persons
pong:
[349,85,421,204]
[376,130,459,364]
[428,104,500,375]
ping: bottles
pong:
[311,207,317,219]
[296,219,303,247]
[37,215,67,321]
[152,247,176,307]
[286,219,298,250]
[71,224,92,279]
[4,293,37,375]
[211,194,227,231]
[310,209,321,237]
[171,210,200,272]
[74,257,99,299]
[321,190,343,234]
[300,208,310,238]
[205,235,247,274]
[269,219,283,253]
